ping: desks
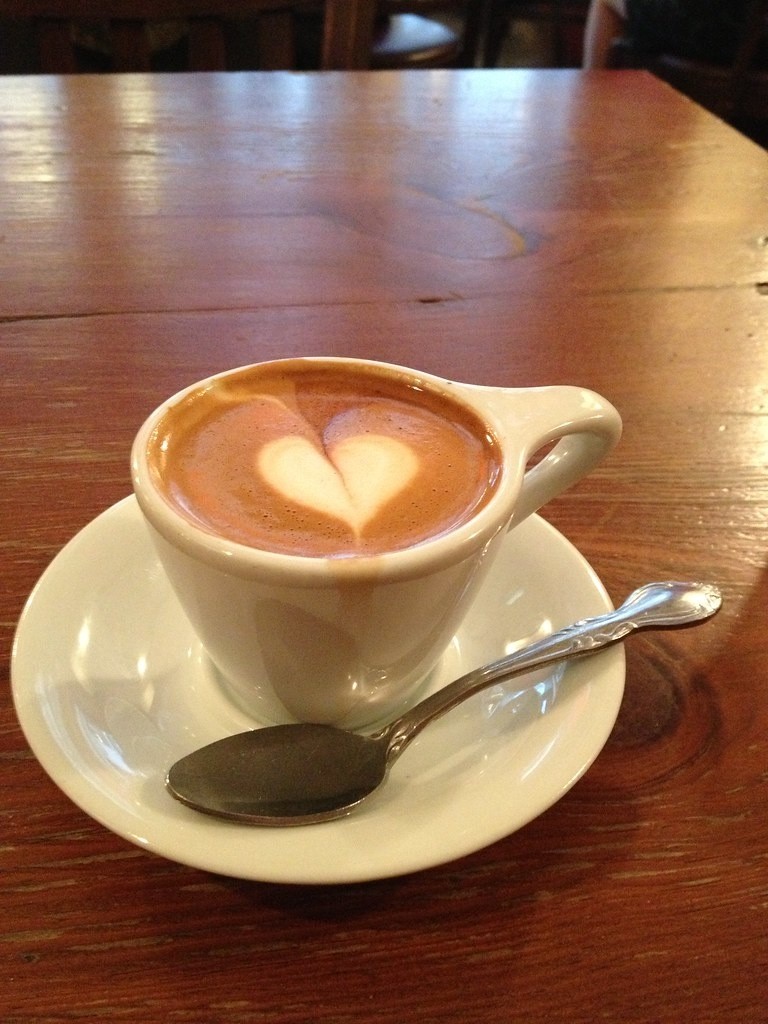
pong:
[0,67,768,1024]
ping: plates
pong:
[11,493,626,879]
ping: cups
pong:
[129,356,622,733]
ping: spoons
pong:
[165,581,723,826]
